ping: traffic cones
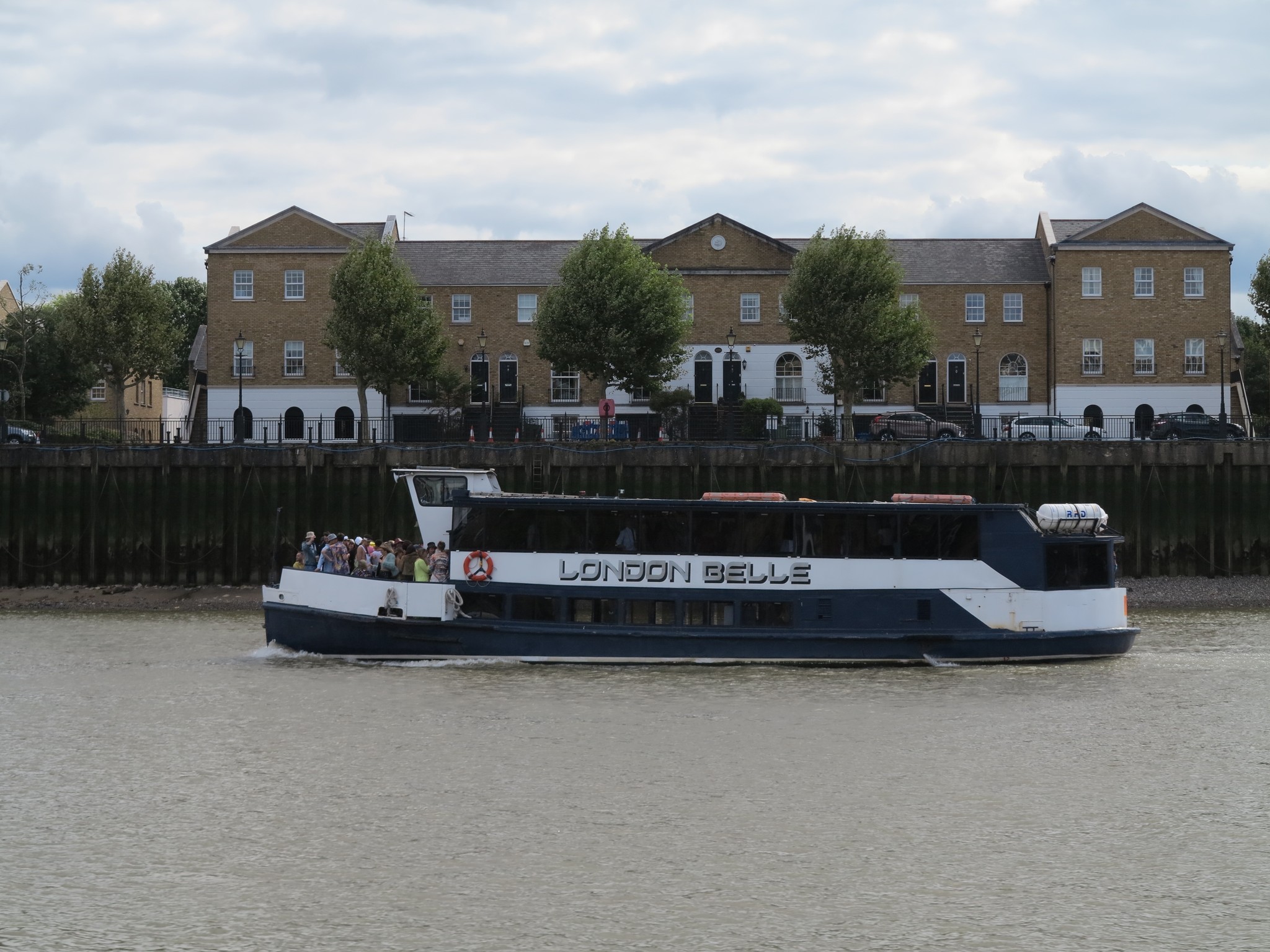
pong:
[468,425,475,442]
[514,428,519,443]
[488,427,494,442]
[539,429,545,442]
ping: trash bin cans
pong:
[840,413,884,441]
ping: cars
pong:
[7,424,37,445]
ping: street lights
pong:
[1215,326,1229,424]
[723,326,738,440]
[234,328,247,445]
[971,326,984,441]
[476,327,490,441]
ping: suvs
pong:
[1148,411,1246,442]
[1000,415,1107,441]
[868,411,966,442]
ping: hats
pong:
[388,541,396,545]
[426,542,438,550]
[395,539,406,543]
[305,531,315,538]
[370,542,376,547]
[380,542,395,554]
[348,539,356,548]
[343,535,350,542]
[355,537,363,545]
[319,537,324,542]
[327,534,337,543]
[373,538,382,545]
[412,544,422,551]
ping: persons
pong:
[617,513,638,554]
[1113,551,1118,573]
[294,530,449,583]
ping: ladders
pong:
[532,452,542,494]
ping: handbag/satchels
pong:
[396,574,409,581]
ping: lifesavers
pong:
[463,551,493,581]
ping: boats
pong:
[258,462,1144,668]
